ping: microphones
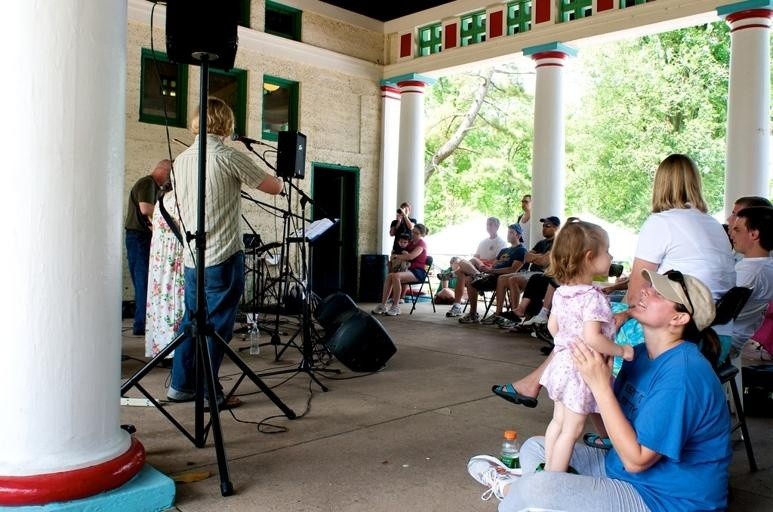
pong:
[231,133,264,145]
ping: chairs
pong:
[399,256,436,314]
[481,262,531,321]
[462,290,488,314]
[713,286,757,474]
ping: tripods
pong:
[120,60,297,496]
[293,242,323,319]
[232,212,288,339]
[245,255,274,319]
[238,193,311,364]
[243,144,342,393]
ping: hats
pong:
[540,216,560,227]
[640,268,716,331]
[508,224,525,243]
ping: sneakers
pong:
[458,312,479,324]
[446,304,461,316]
[523,316,547,326]
[467,455,522,501]
[500,319,518,329]
[371,304,387,314]
[481,313,497,324]
[385,306,401,316]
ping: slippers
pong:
[583,432,612,449]
[500,310,522,322]
[491,384,538,408]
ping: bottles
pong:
[250,324,259,354]
[500,431,521,469]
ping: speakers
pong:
[358,255,388,303]
[165,1,239,71]
[314,291,397,375]
[277,131,307,179]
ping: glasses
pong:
[542,224,550,228]
[521,199,528,204]
[663,269,695,316]
[410,231,419,235]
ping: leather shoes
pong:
[200,397,242,412]
[163,384,187,406]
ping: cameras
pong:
[397,209,402,214]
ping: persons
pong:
[539,218,634,475]
[497,216,560,334]
[445,217,508,317]
[144,187,186,369]
[433,257,467,305]
[516,196,530,250]
[386,234,411,304]
[490,154,734,451]
[371,223,429,315]
[702,206,772,365]
[467,270,731,510]
[124,159,171,336]
[166,96,283,410]
[390,201,417,305]
[727,196,772,238]
[459,224,529,324]
[488,253,511,274]
